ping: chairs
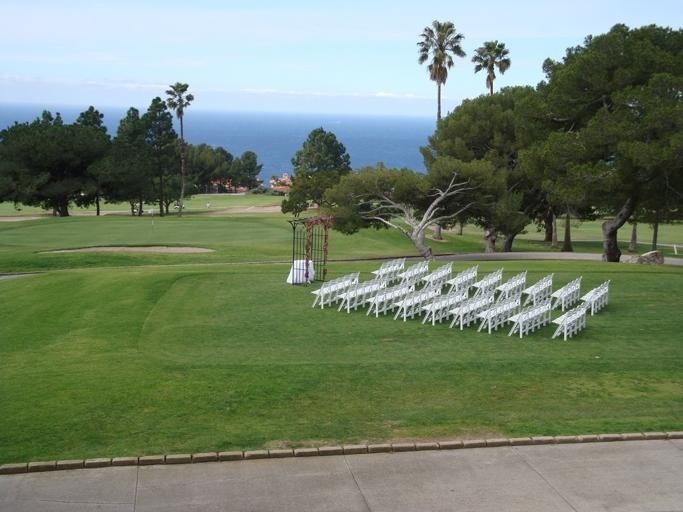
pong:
[311,256,611,342]
